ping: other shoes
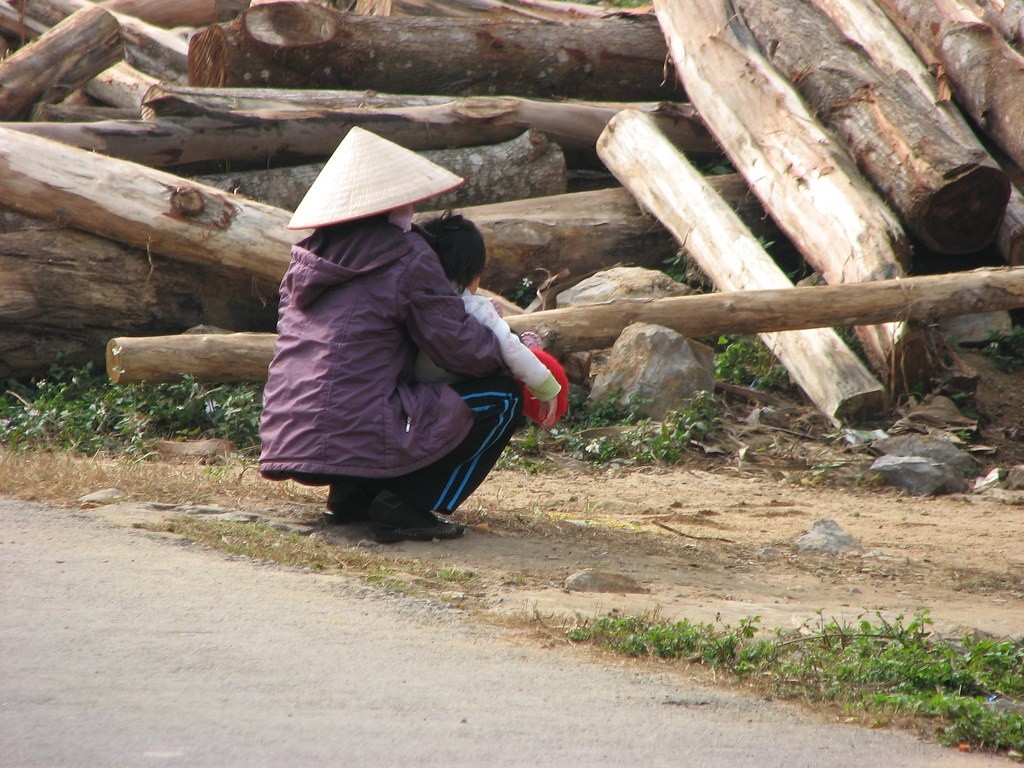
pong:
[521,332,543,351]
[486,295,502,318]
[370,514,463,544]
[322,502,376,525]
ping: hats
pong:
[287,126,466,232]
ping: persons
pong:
[258,126,522,541]
[411,211,570,427]
